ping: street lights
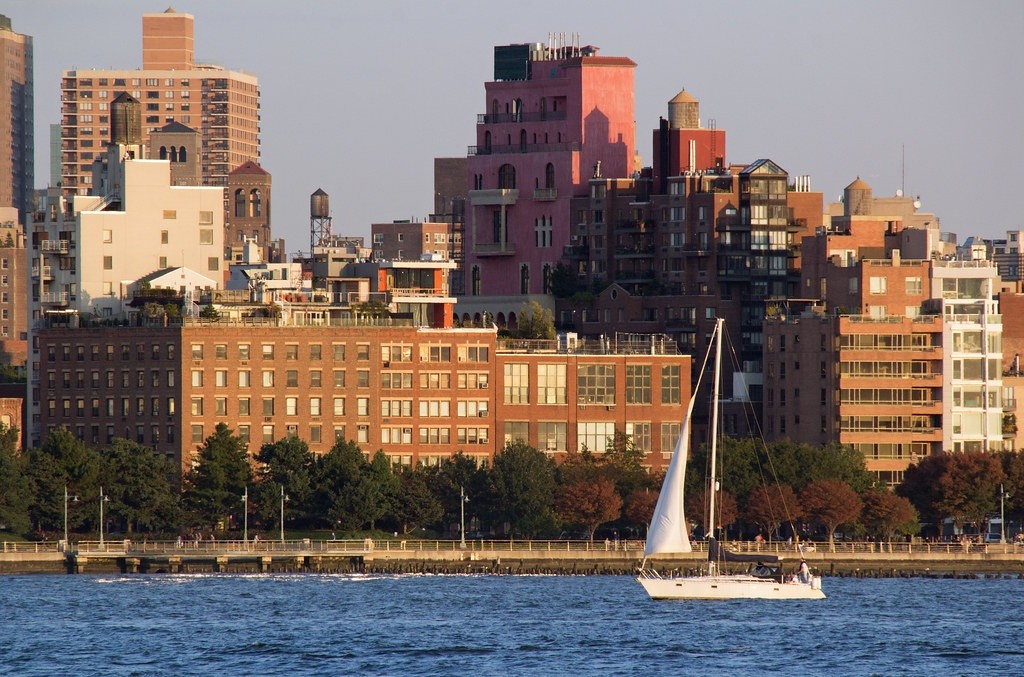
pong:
[1000,492,1009,543]
[280,495,289,540]
[100,495,110,543]
[460,496,471,547]
[64,495,80,541]
[241,496,247,539]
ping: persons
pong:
[924,534,984,553]
[864,535,880,552]
[691,540,698,551]
[364,533,370,549]
[1013,532,1024,554]
[785,535,816,552]
[174,532,217,548]
[755,534,767,553]
[330,532,338,551]
[797,559,808,579]
[253,532,260,551]
[604,538,644,551]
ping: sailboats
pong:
[635,316,827,598]
